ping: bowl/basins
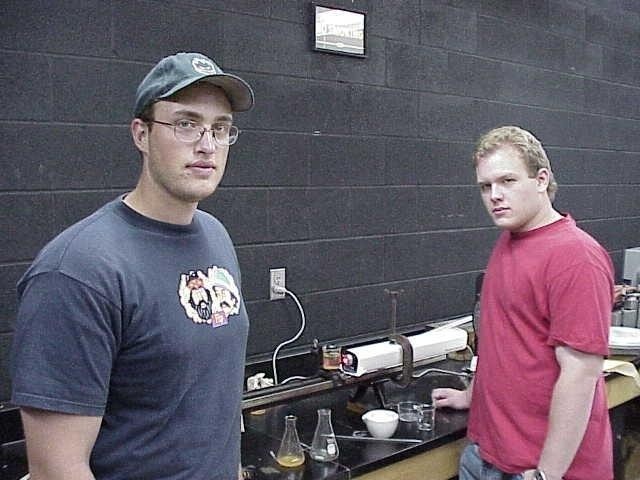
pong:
[361,408,399,439]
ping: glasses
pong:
[147,118,241,148]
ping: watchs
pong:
[532,464,547,480]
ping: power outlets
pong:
[270,268,288,301]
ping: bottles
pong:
[309,408,340,463]
[275,415,306,468]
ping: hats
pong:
[130,50,255,118]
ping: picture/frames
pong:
[310,2,371,62]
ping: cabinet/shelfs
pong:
[605,365,640,409]
[350,437,467,480]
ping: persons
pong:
[7,52,255,480]
[431,124,617,479]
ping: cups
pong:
[398,400,421,423]
[611,308,637,327]
[413,404,436,432]
[322,344,341,370]
